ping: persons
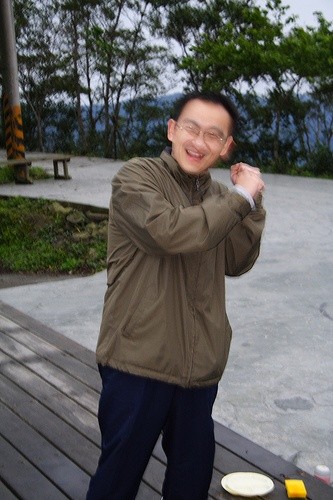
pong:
[84,91,268,500]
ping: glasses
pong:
[175,121,227,144]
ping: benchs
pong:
[0,156,72,184]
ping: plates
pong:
[221,472,237,494]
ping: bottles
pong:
[314,465,330,483]
